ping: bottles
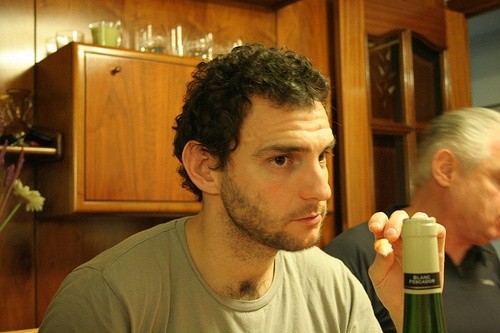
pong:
[402,218,447,333]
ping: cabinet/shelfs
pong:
[35,40,208,222]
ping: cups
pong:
[134,23,168,54]
[171,24,214,59]
[88,20,126,49]
[56,30,84,49]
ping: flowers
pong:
[0,178,46,233]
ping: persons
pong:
[323,106,500,333]
[38,42,446,333]
